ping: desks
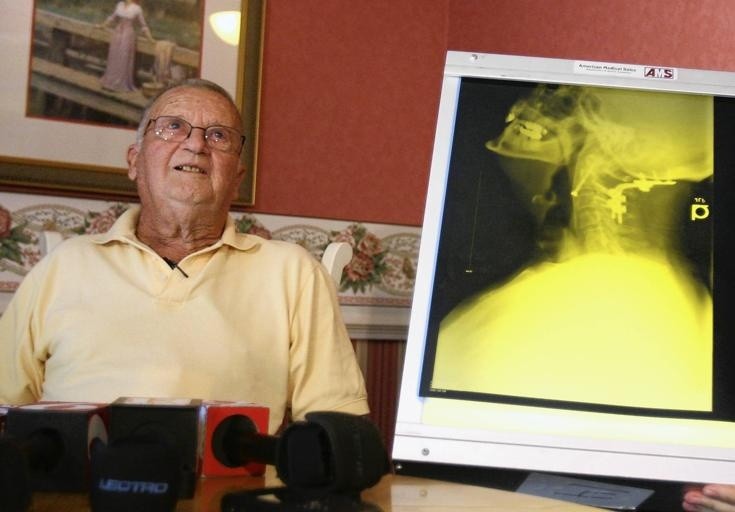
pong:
[0,459,617,512]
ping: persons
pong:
[0,78,370,478]
[683,484,735,511]
[93,0,156,95]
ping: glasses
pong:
[144,115,246,156]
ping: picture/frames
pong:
[0,0,268,208]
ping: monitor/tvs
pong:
[390,52,735,512]
